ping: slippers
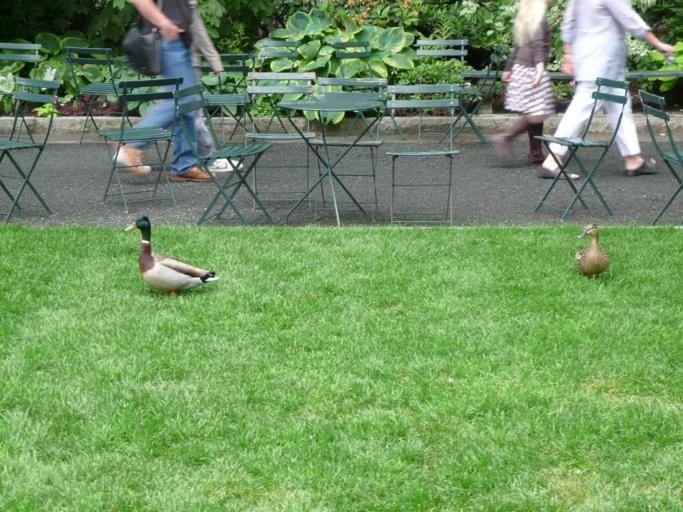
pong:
[626,156,659,176]
[537,164,580,180]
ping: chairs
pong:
[638,89,681,227]
[0,31,494,230]
[534,77,631,223]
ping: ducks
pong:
[123,215,221,296]
[574,222,610,281]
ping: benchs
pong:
[461,70,681,114]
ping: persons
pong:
[538,0,676,181]
[112,0,215,183]
[170,0,250,174]
[486,0,556,167]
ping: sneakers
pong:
[201,159,242,173]
[112,145,151,175]
[169,166,214,183]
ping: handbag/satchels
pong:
[123,23,165,76]
